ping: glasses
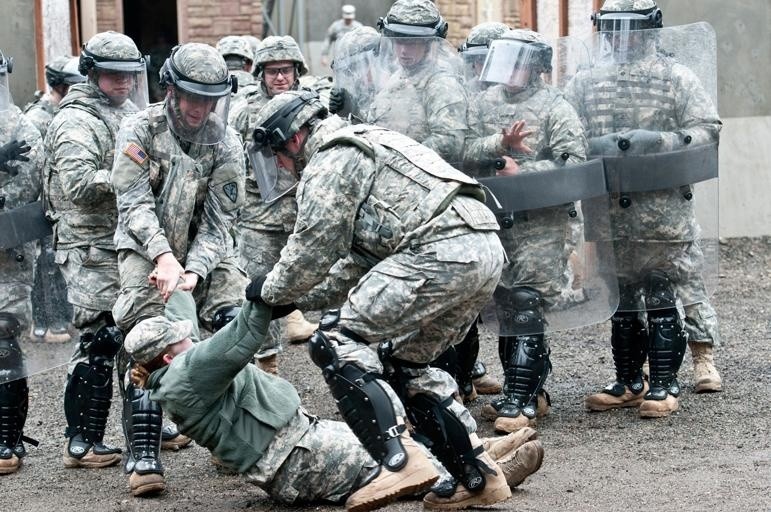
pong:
[262,65,295,76]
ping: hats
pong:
[124,315,193,365]
[341,4,357,19]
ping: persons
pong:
[363,1,476,402]
[247,90,515,508]
[214,35,319,343]
[125,316,545,505]
[319,5,361,68]
[23,55,87,345]
[465,28,587,435]
[562,0,722,420]
[222,36,316,375]
[0,52,45,475]
[460,22,512,91]
[44,31,146,468]
[640,249,722,392]
[111,41,256,497]
[328,27,502,395]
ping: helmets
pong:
[250,35,309,79]
[0,48,14,115]
[263,64,297,77]
[331,26,382,71]
[159,42,238,145]
[245,86,331,204]
[464,20,511,54]
[43,55,88,88]
[216,35,255,62]
[78,30,149,115]
[375,0,449,77]
[591,0,665,64]
[478,28,553,89]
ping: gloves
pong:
[245,274,267,302]
[589,131,623,155]
[1,139,32,176]
[619,129,662,155]
[273,302,299,320]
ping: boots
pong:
[160,434,191,453]
[584,379,653,410]
[0,452,26,475]
[28,325,72,345]
[473,375,504,394]
[256,358,279,377]
[496,440,544,488]
[63,439,122,469]
[284,309,320,344]
[493,412,537,433]
[126,472,167,496]
[345,417,440,512]
[638,389,679,418]
[687,339,723,393]
[423,433,513,509]
[480,426,536,461]
[481,392,551,422]
